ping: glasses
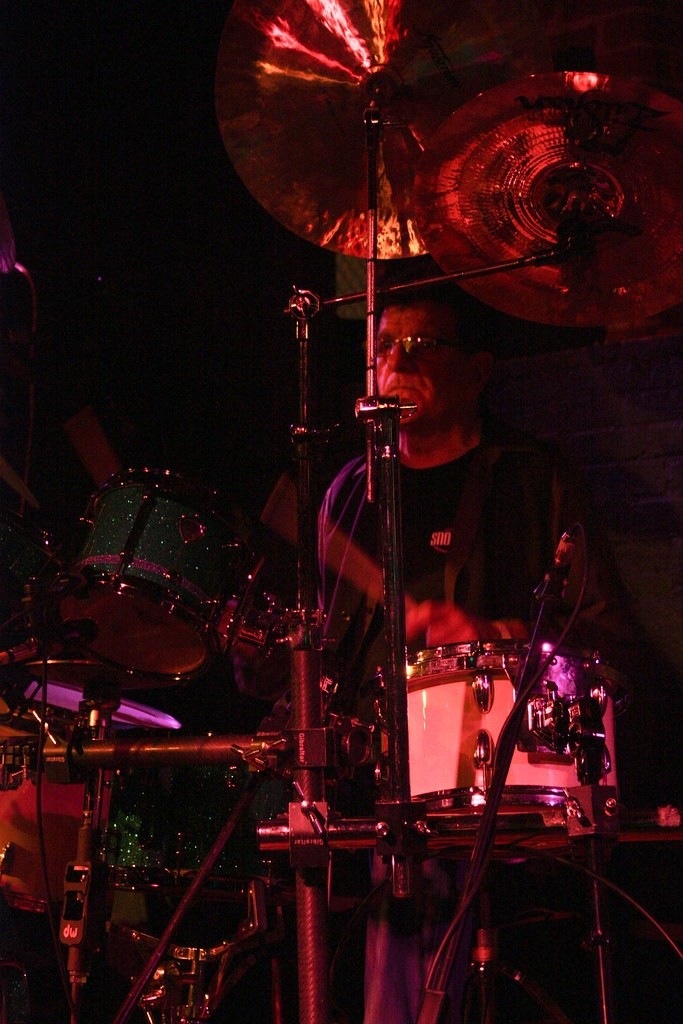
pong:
[378,330,460,358]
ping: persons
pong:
[236,288,640,1023]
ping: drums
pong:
[58,462,245,677]
[2,713,115,918]
[373,636,619,833]
[218,536,374,693]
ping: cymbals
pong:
[215,0,550,262]
[415,68,682,329]
